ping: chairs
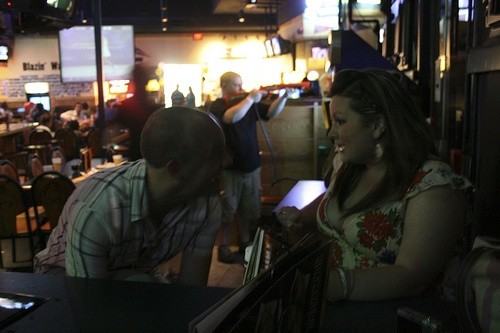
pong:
[454,245,500,333]
[0,128,88,273]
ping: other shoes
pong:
[217,245,244,263]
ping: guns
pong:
[223,81,311,106]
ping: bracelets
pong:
[336,267,355,301]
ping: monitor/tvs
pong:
[264,36,291,57]
[0,44,9,62]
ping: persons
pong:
[35,106,233,287]
[108,61,162,166]
[186,86,195,107]
[210,72,299,264]
[277,68,477,333]
[171,84,185,107]
[0,98,129,161]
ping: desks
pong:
[0,123,39,137]
[0,269,237,333]
[21,162,121,186]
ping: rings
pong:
[281,212,287,213]
